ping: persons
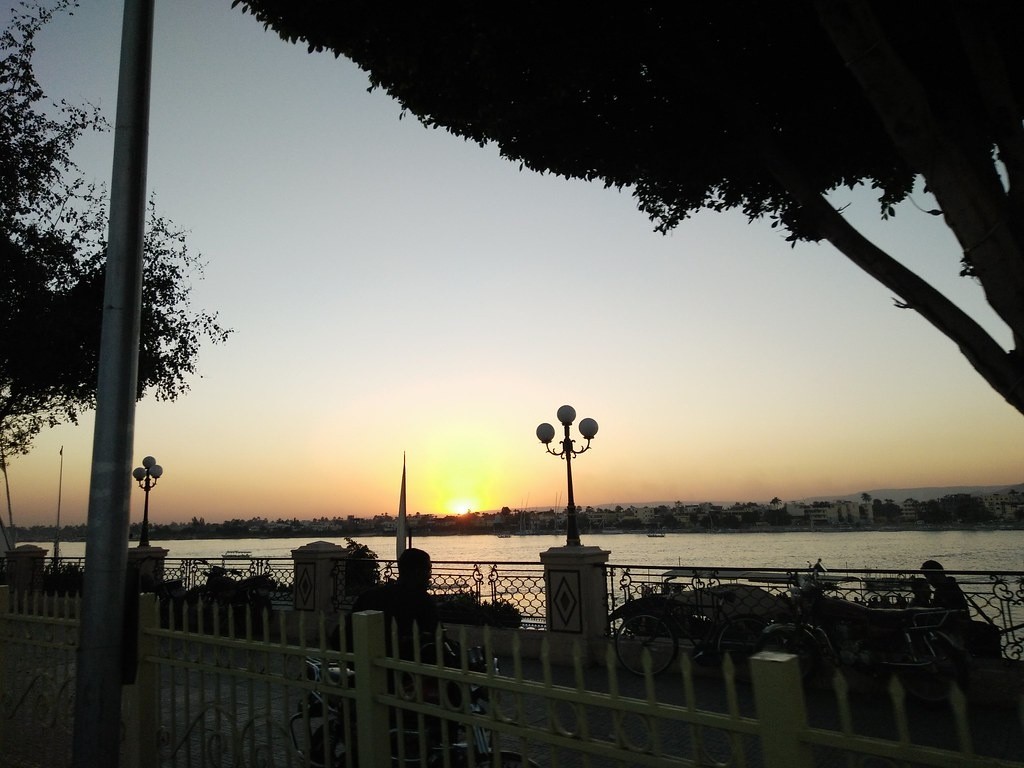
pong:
[387,549,442,654]
[907,560,970,619]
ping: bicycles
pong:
[615,576,789,688]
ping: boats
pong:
[648,533,665,537]
[862,574,929,587]
[496,534,511,538]
[222,549,253,559]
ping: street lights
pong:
[130,455,164,546]
[534,403,599,546]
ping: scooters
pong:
[155,556,272,640]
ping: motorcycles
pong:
[287,625,541,768]
